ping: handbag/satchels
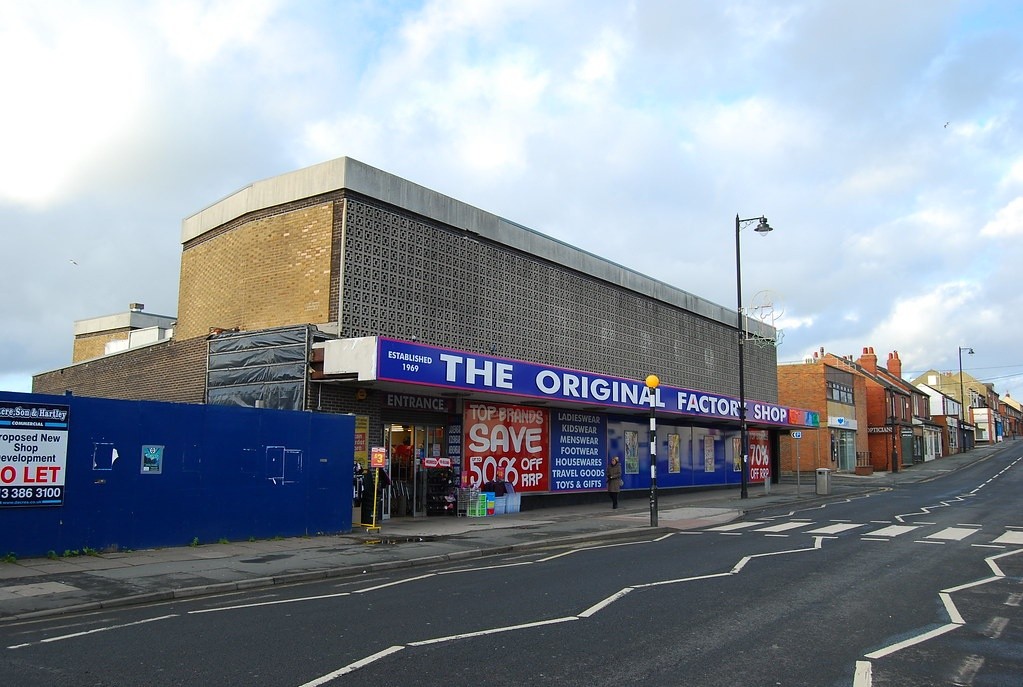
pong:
[618,479,624,486]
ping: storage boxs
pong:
[504,492,522,514]
[494,496,506,514]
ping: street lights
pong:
[959,347,976,453]
[646,375,660,526]
[736,215,774,496]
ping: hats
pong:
[612,456,619,461]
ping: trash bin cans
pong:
[816,468,830,494]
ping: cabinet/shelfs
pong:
[390,442,454,516]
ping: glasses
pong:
[611,459,616,462]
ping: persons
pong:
[606,456,623,507]
